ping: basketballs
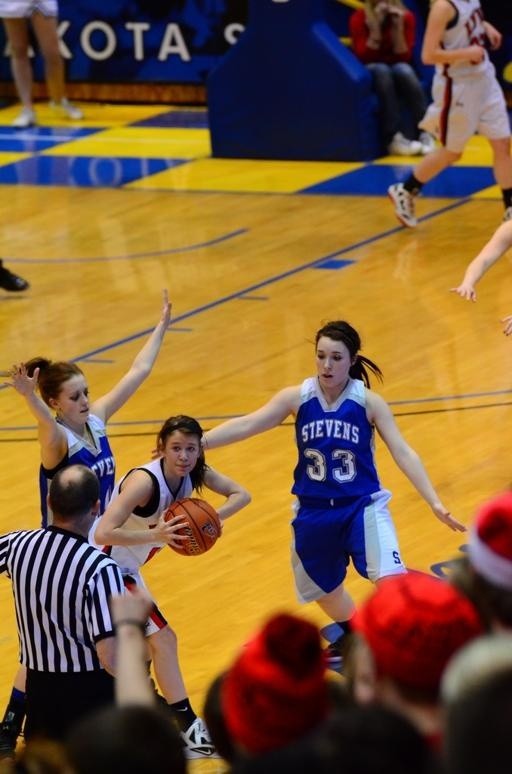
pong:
[165,498,221,556]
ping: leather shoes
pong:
[0,260,26,291]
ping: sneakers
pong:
[50,100,84,118]
[387,132,435,157]
[177,717,213,759]
[11,108,37,128]
[387,182,416,229]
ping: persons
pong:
[1,288,178,760]
[1,0,89,130]
[1,256,33,294]
[342,0,436,157]
[1,704,194,773]
[450,202,511,340]
[2,460,154,745]
[86,411,256,761]
[383,0,512,236]
[200,483,512,774]
[151,320,470,664]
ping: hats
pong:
[465,491,512,588]
[349,573,483,688]
[220,613,330,752]
